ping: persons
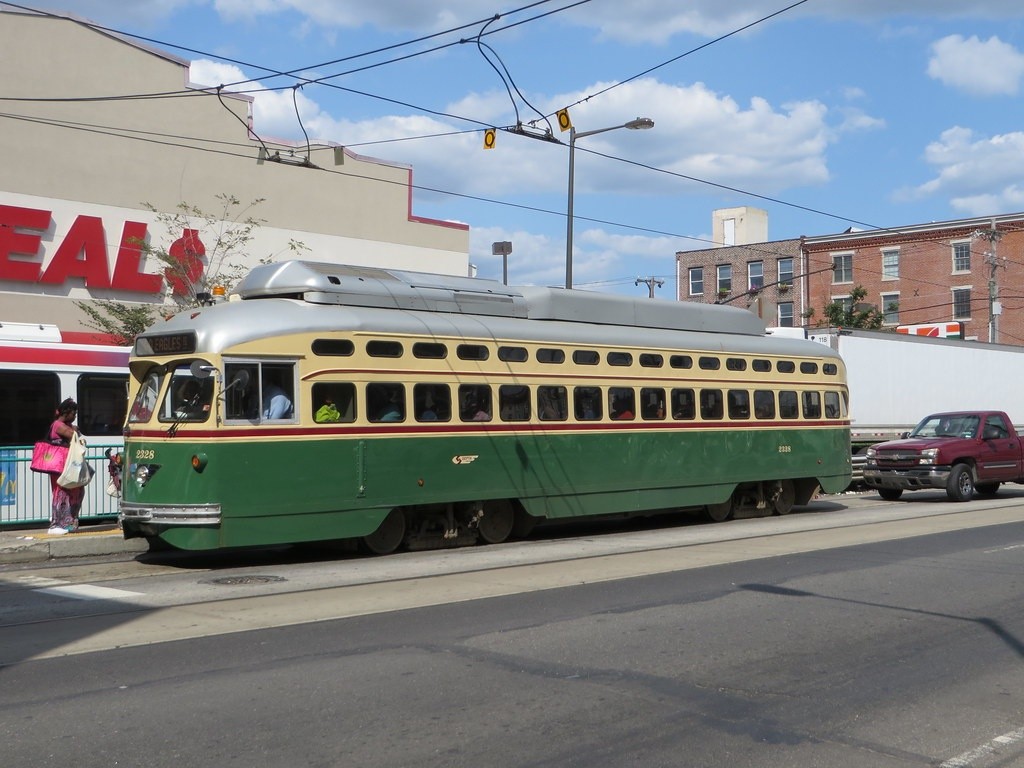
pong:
[246,369,293,421]
[370,404,402,420]
[611,395,632,418]
[418,408,438,420]
[464,394,489,419]
[315,397,340,422]
[47,397,93,535]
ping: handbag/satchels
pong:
[55,431,93,490]
[30,422,69,474]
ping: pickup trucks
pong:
[862,411,1024,502]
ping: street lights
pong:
[566,118,655,289]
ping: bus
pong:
[117,257,851,557]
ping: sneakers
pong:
[48,527,68,534]
